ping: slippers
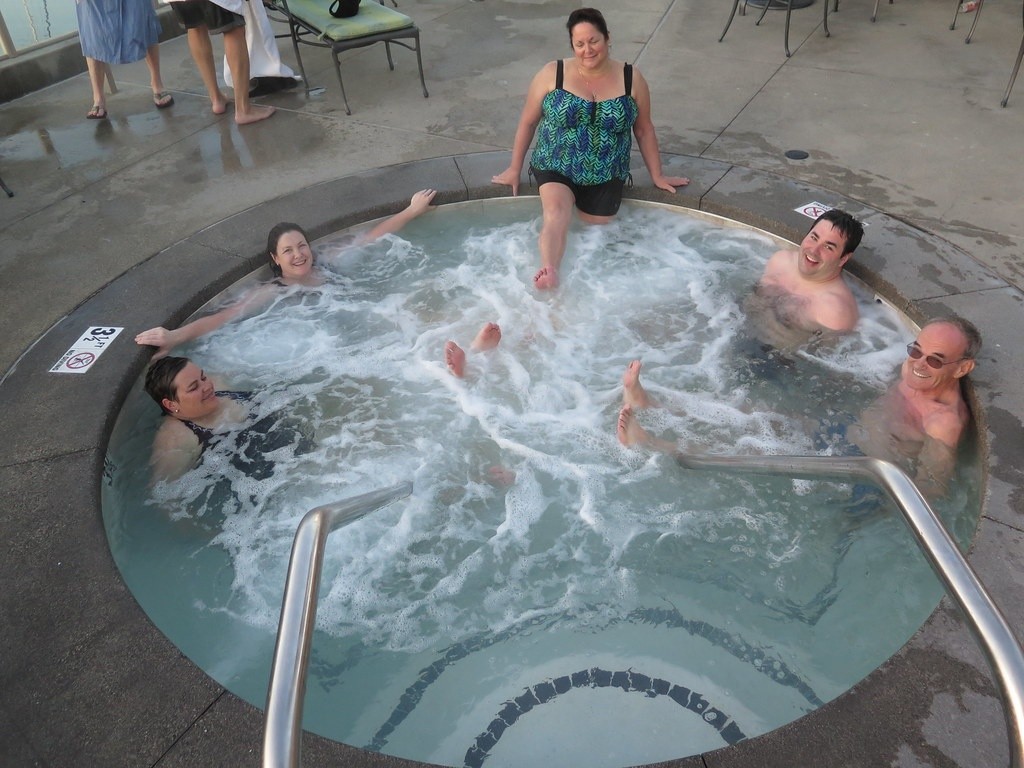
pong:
[152,90,174,109]
[85,105,108,119]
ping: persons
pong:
[617,317,982,444]
[75,0,174,118]
[165,0,275,122]
[135,189,501,375]
[770,209,865,330]
[145,356,218,446]
[491,8,690,287]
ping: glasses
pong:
[906,341,969,369]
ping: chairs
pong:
[262,0,428,116]
